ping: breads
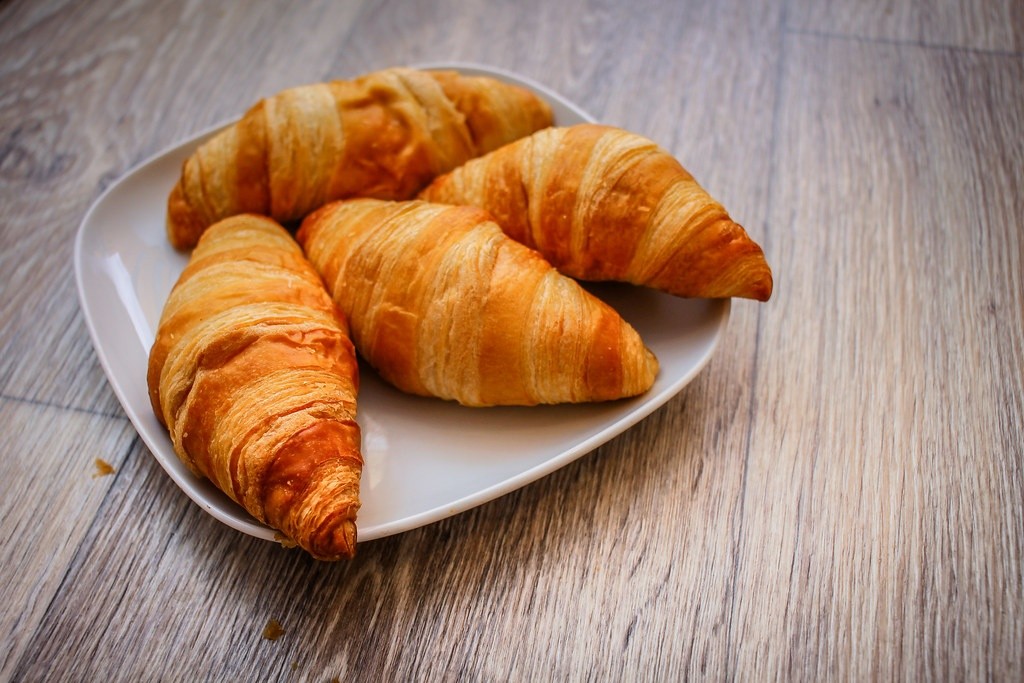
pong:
[163,67,556,251]
[146,212,363,563]
[417,123,773,303]
[294,197,658,407]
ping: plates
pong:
[74,61,732,542]
